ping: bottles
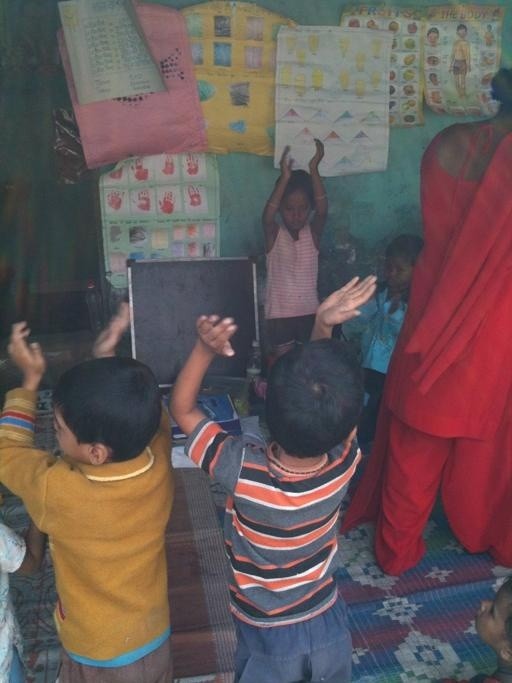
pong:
[245,341,262,386]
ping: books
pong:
[170,445,201,469]
[168,435,242,447]
[164,392,243,440]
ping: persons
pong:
[424,24,440,47]
[167,271,380,682]
[1,495,48,683]
[435,575,512,683]
[246,134,331,405]
[448,19,475,102]
[340,55,512,577]
[1,296,180,682]
[342,230,426,446]
[479,22,496,45]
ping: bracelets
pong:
[312,193,329,201]
[265,200,282,210]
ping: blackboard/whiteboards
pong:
[125,256,261,389]
